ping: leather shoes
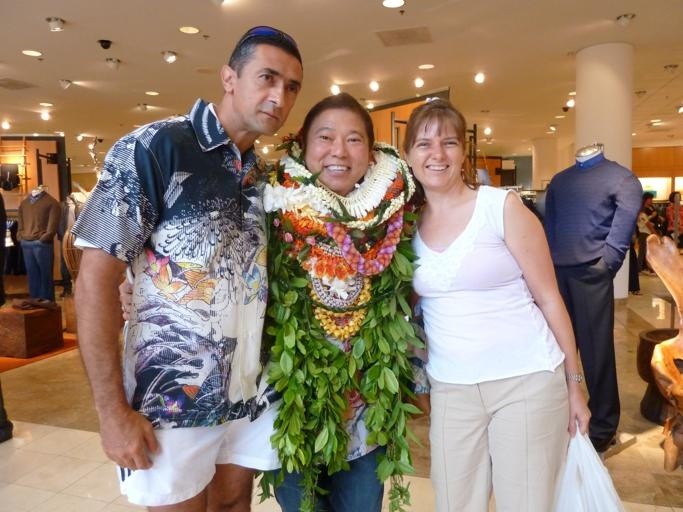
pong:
[12,300,60,313]
[589,431,618,452]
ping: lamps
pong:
[43,17,177,90]
[88,136,98,159]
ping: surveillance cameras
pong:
[97,137,104,143]
[96,35,113,50]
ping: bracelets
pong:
[566,373,583,383]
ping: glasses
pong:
[229,25,299,68]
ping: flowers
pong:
[254,131,425,512]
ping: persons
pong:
[666,191,683,255]
[401,98,592,512]
[0,193,14,443]
[70,25,303,512]
[636,212,663,272]
[264,91,422,512]
[546,144,643,453]
[16,188,83,306]
[630,243,643,295]
[642,192,664,235]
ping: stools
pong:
[0,303,63,359]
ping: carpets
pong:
[0,323,128,434]
